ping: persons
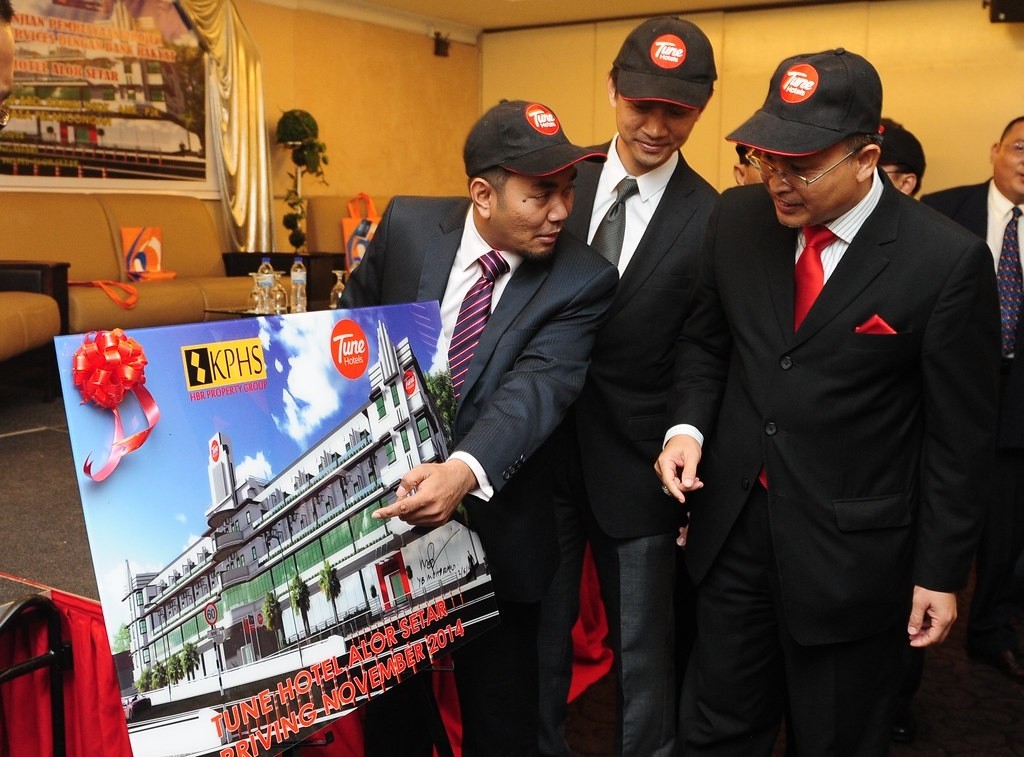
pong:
[734,117,1024,684]
[565,14,721,757]
[0,1,18,133]
[654,47,1000,757]
[335,98,618,757]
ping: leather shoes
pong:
[961,634,1024,684]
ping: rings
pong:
[661,485,671,497]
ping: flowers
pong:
[72,328,161,481]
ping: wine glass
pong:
[249,271,288,316]
[329,269,347,310]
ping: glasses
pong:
[1010,141,1024,152]
[745,148,859,188]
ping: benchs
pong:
[0,189,294,403]
[306,193,390,253]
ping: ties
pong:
[793,224,840,335]
[996,206,1023,356]
[448,250,510,400]
[589,179,639,268]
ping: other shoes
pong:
[889,705,917,746]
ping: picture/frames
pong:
[0,0,224,201]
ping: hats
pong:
[464,100,607,177]
[613,15,717,110]
[726,47,882,157]
[879,117,926,175]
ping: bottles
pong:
[289,257,306,314]
[257,258,274,273]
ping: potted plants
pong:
[221,106,347,312]
